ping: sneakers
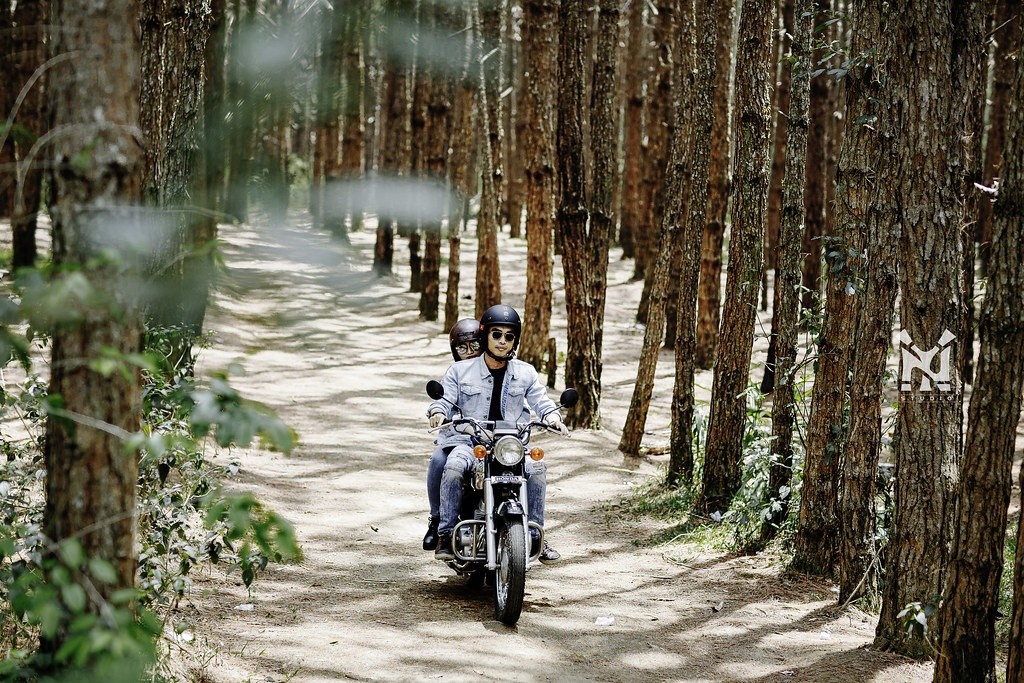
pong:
[434,534,456,560]
[422,515,441,550]
[538,540,562,564]
[529,538,541,562]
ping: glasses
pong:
[490,331,516,342]
[455,339,482,355]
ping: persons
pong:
[422,305,571,566]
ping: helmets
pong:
[479,305,521,351]
[449,318,484,362]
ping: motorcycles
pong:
[426,381,578,625]
